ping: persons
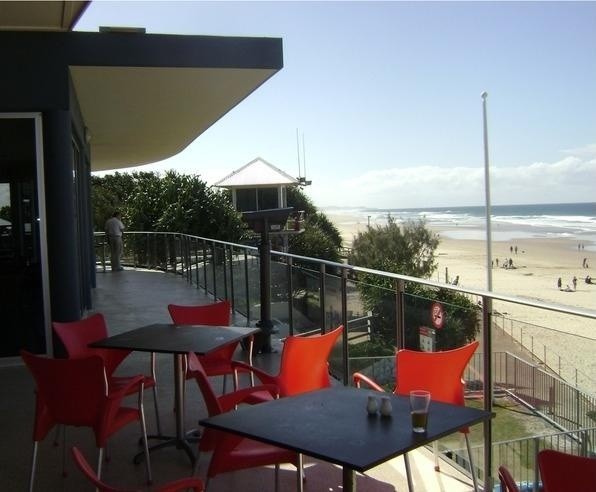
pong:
[515,247,518,253]
[492,256,513,270]
[558,278,561,290]
[573,276,577,290]
[582,258,586,268]
[582,244,585,250]
[585,275,589,284]
[577,243,581,251]
[565,285,572,291]
[588,277,593,283]
[586,263,589,268]
[104,211,125,271]
[511,246,513,253]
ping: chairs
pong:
[70,444,203,492]
[53,312,162,462]
[350,340,480,491]
[168,300,256,412]
[185,349,305,492]
[19,348,153,491]
[498,450,596,492]
[231,325,344,410]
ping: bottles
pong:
[381,396,392,417]
[367,395,378,417]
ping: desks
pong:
[199,383,496,492]
[87,322,262,471]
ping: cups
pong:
[410,391,431,433]
